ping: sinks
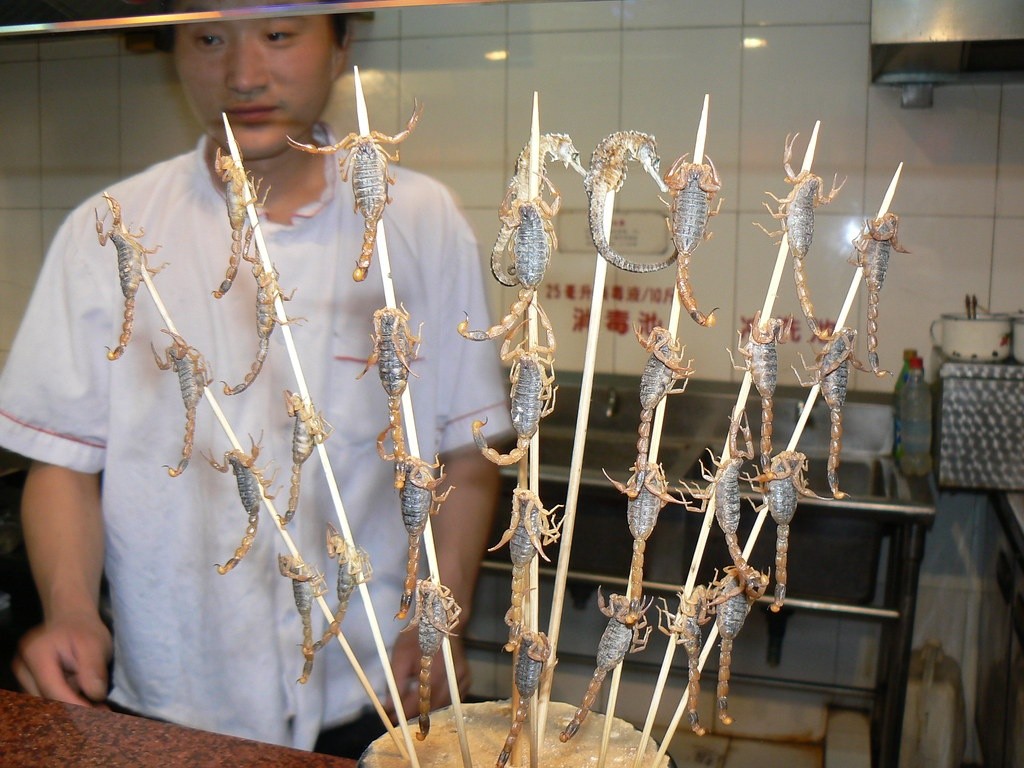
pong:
[496,428,680,499]
[683,448,936,537]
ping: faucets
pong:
[605,387,619,417]
[796,402,807,432]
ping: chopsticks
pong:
[965,295,977,320]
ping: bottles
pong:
[899,355,933,476]
[893,347,918,459]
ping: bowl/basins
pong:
[930,310,1012,362]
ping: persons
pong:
[0,0,511,759]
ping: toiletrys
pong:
[892,347,933,477]
